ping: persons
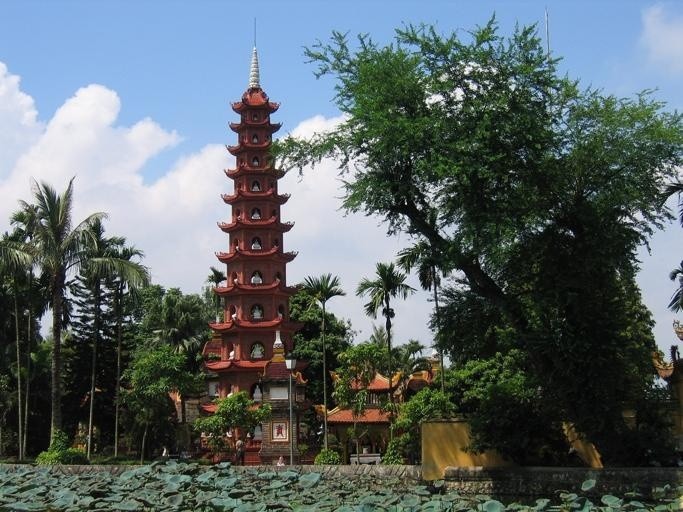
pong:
[277,456,286,466]
[236,436,245,466]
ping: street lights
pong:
[284,353,300,467]
